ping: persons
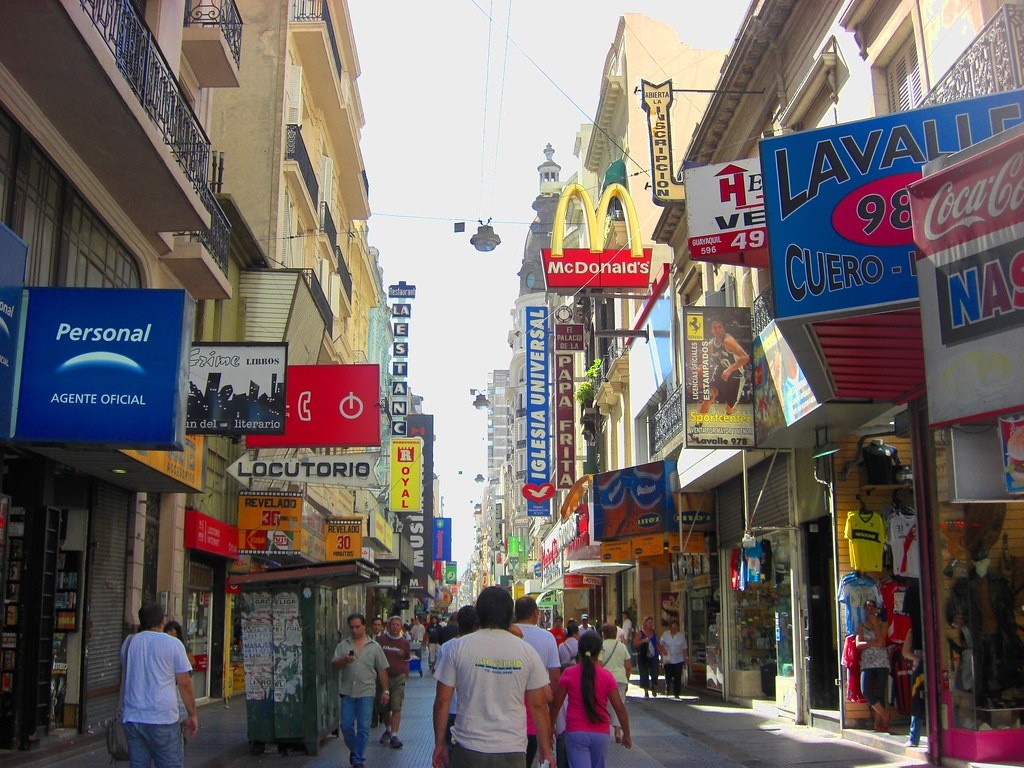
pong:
[632,616,661,698]
[372,610,441,748]
[164,620,197,745]
[900,627,926,747]
[659,621,690,698]
[433,597,633,768]
[694,314,749,427]
[328,613,391,768]
[119,601,199,768]
[946,553,1013,701]
[430,585,558,768]
[551,629,632,768]
[856,599,893,732]
[944,612,976,691]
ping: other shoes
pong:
[881,712,893,731]
[616,734,621,743]
[380,731,392,743]
[370,719,378,728]
[652,688,657,697]
[644,694,649,700]
[675,696,680,700]
[354,763,365,768]
[874,727,882,732]
[665,685,671,695]
[379,713,383,724]
[904,740,917,747]
[390,736,403,748]
[349,751,355,765]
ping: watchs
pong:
[384,691,389,694]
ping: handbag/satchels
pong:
[107,717,131,761]
[646,640,655,658]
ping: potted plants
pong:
[576,383,593,416]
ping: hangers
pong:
[859,499,871,514]
[890,494,914,516]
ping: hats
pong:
[622,610,630,616]
[581,614,589,621]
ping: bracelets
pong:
[686,657,688,658]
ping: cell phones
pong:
[350,650,354,656]
